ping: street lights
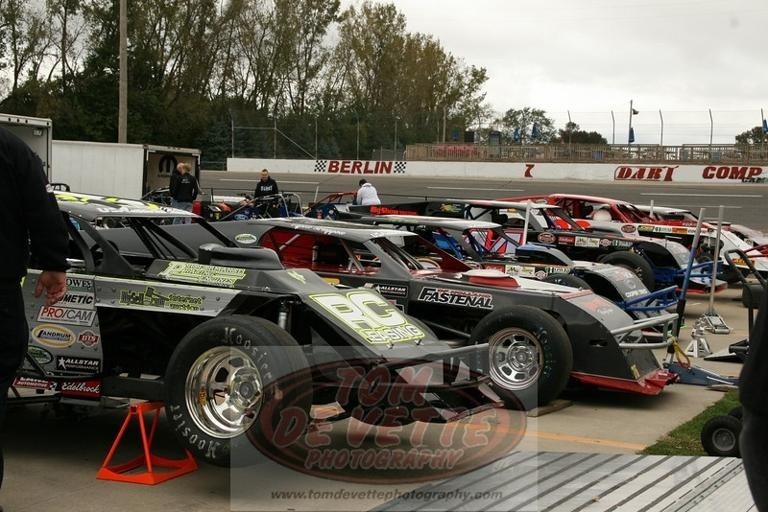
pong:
[628,99,639,145]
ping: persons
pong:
[357,180,381,206]
[737,284,768,512]
[0,129,71,388]
[254,170,278,198]
[169,162,204,224]
